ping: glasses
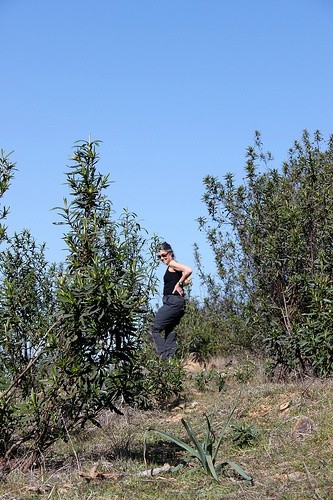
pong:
[158,252,169,259]
